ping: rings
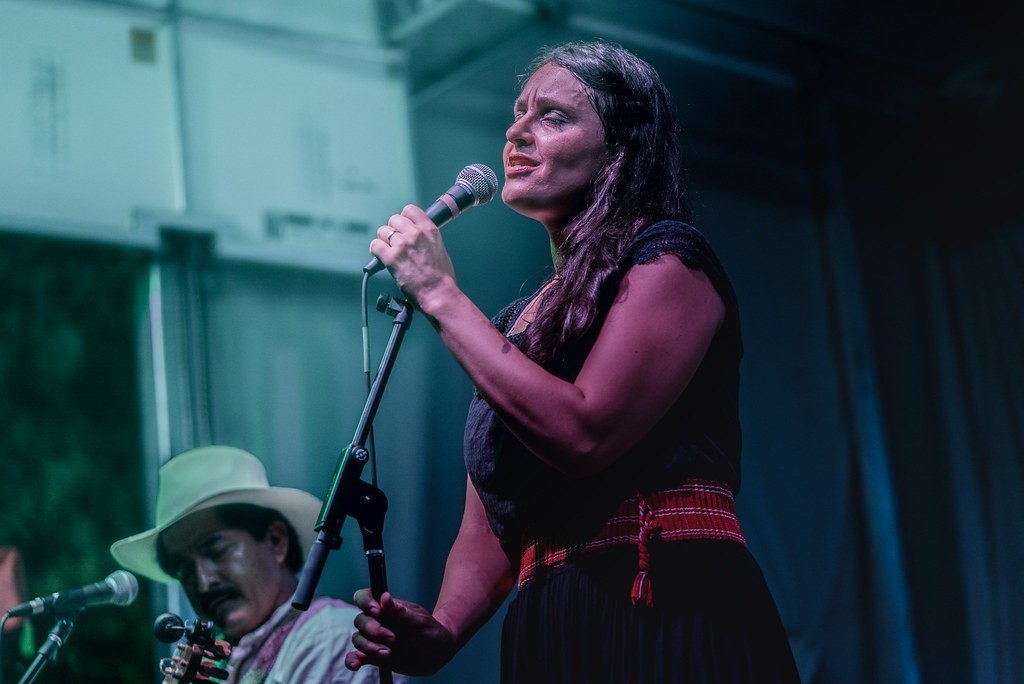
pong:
[387,230,401,247]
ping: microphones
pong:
[8,569,139,618]
[363,164,498,276]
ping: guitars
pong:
[149,610,230,684]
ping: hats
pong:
[110,445,325,586]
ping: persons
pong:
[342,37,804,684]
[109,444,409,684]
